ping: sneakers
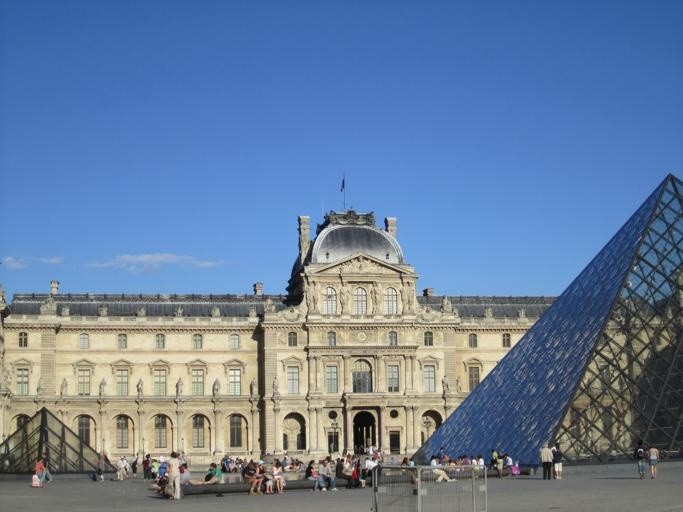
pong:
[320,487,339,492]
[249,489,284,496]
[640,476,657,480]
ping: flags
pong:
[340,178,345,192]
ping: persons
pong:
[306,445,386,492]
[198,454,306,496]
[633,439,660,480]
[153,450,190,500]
[390,448,520,483]
[116,453,160,485]
[31,456,53,486]
[539,442,563,480]
